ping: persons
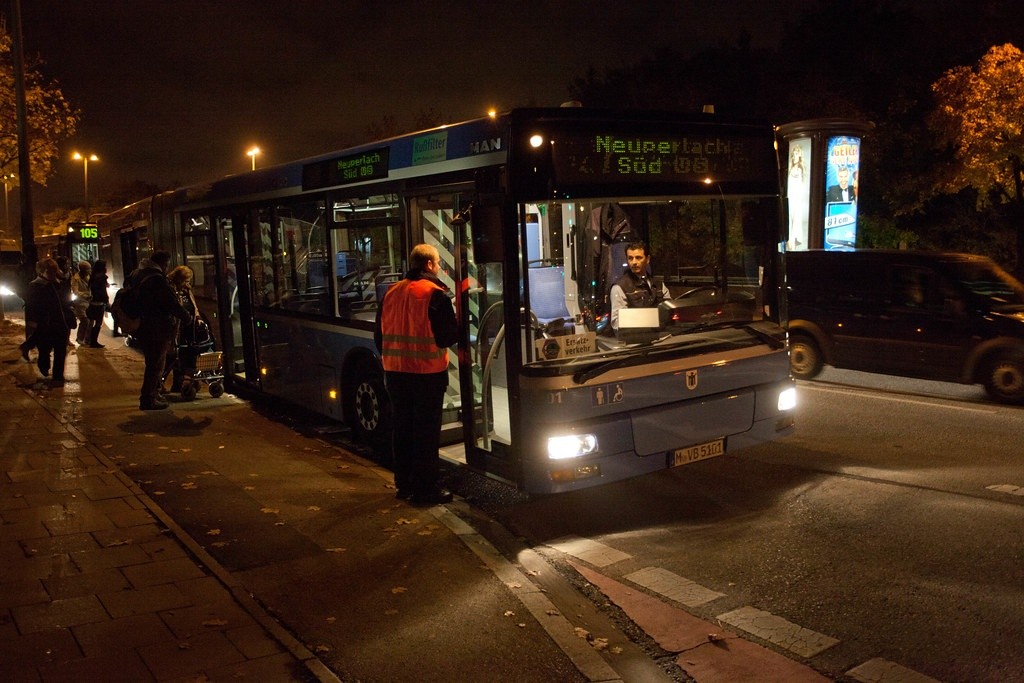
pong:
[130,249,193,410]
[826,166,855,202]
[162,266,203,392]
[20,256,74,381]
[609,240,671,337]
[374,245,458,504]
[88,260,109,348]
[68,261,93,346]
[788,144,806,251]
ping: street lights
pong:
[245,144,264,170]
[71,151,100,222]
[4,173,14,235]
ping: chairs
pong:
[525,256,576,332]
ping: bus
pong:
[34,221,98,298]
[97,99,792,495]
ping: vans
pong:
[0,239,22,306]
[760,251,1024,404]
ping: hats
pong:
[79,261,91,269]
[149,249,171,266]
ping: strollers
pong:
[175,314,225,400]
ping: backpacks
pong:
[109,273,162,332]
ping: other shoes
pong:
[156,392,168,402]
[84,340,91,344]
[19,343,30,361]
[396,489,452,503]
[113,333,121,336]
[170,383,185,392]
[122,333,128,337]
[90,342,105,348]
[37,359,48,376]
[161,387,170,394]
[76,338,83,344]
[139,396,169,410]
[52,375,64,381]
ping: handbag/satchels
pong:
[194,320,213,354]
